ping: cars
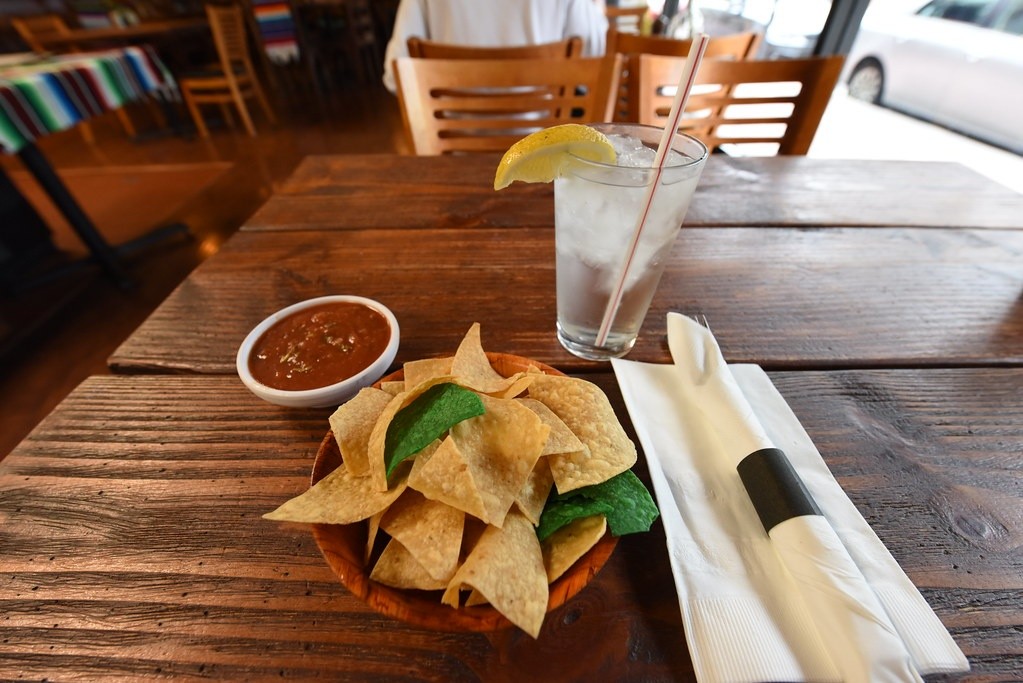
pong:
[841,0,1023,157]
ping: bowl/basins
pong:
[308,352,622,630]
[235,295,399,407]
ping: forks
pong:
[689,315,708,337]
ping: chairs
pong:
[0,5,845,157]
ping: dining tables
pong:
[0,156,1023,683]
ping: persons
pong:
[383,0,609,156]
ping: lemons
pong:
[494,124,619,189]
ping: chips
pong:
[260,322,660,641]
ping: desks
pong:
[0,49,165,146]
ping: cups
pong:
[555,124,709,362]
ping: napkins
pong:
[610,357,970,683]
